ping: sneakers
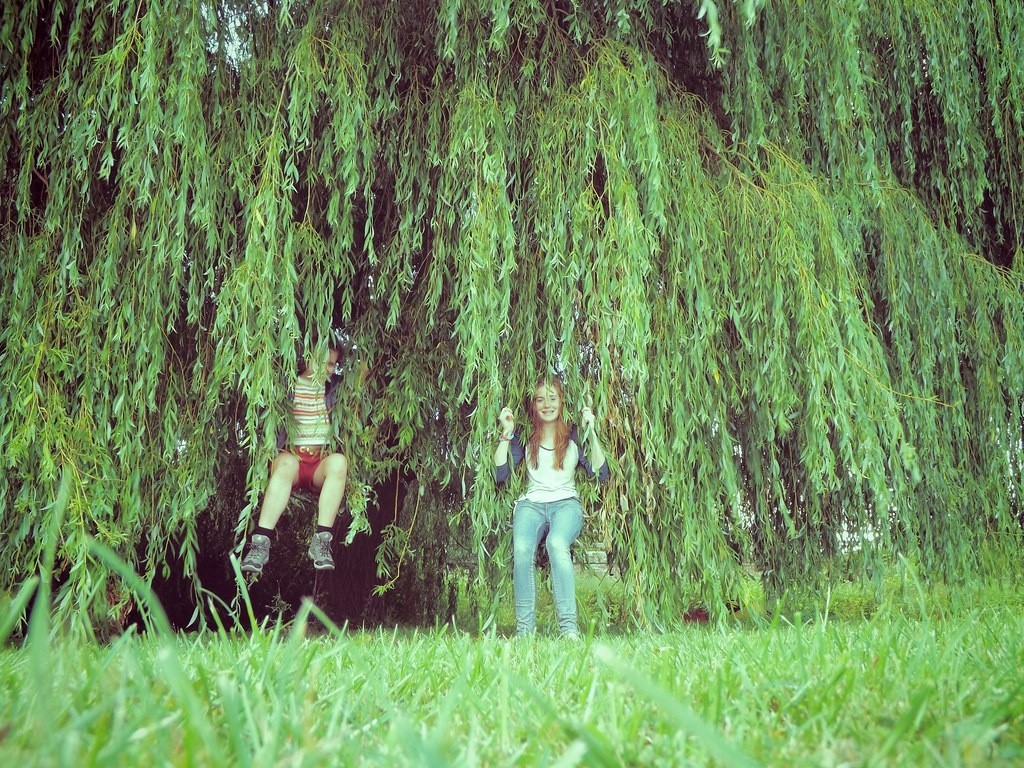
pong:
[308,532,335,572]
[240,534,271,572]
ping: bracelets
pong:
[499,434,514,441]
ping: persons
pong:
[493,377,608,647]
[223,335,391,572]
